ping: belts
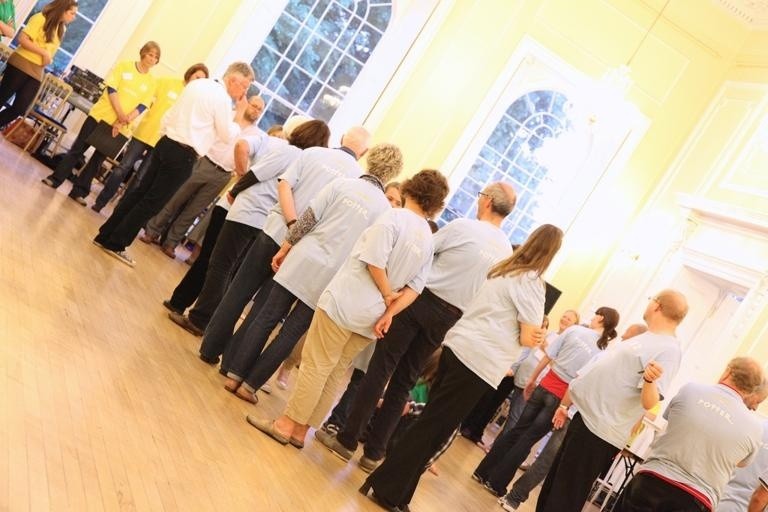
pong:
[203,155,231,174]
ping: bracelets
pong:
[641,374,653,384]
[559,403,569,413]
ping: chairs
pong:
[1,71,74,160]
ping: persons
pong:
[91,62,649,511]
[43,42,161,207]
[0,1,79,129]
[612,352,768,512]
[0,1,17,50]
[534,291,687,509]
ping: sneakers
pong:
[163,297,520,511]
[42,176,196,269]
